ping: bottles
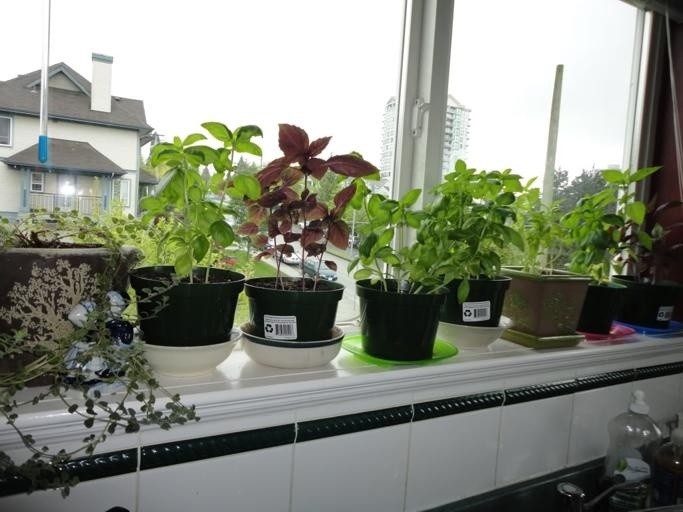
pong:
[655,411,683,506]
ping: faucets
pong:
[555,479,647,511]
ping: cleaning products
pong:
[649,413,683,507]
[603,390,662,511]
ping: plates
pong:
[506,329,584,348]
[438,316,513,346]
[341,335,458,365]
[128,328,238,375]
[577,324,635,341]
[240,324,343,370]
[616,320,682,338]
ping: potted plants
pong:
[0,206,201,500]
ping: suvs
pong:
[299,259,337,281]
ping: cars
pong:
[348,232,359,247]
[258,243,274,251]
[298,221,311,230]
[273,250,301,265]
